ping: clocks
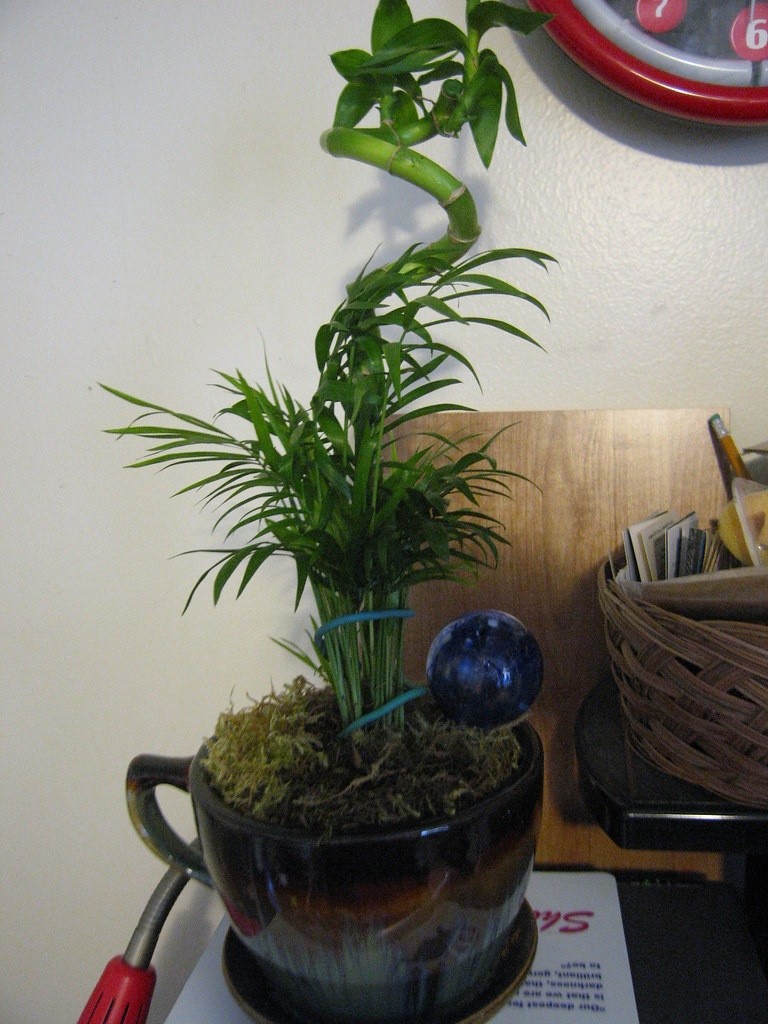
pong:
[528,0,768,128]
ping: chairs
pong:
[380,397,729,883]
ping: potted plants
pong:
[96,0,558,1024]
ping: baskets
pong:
[596,529,768,809]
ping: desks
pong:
[487,862,768,1024]
